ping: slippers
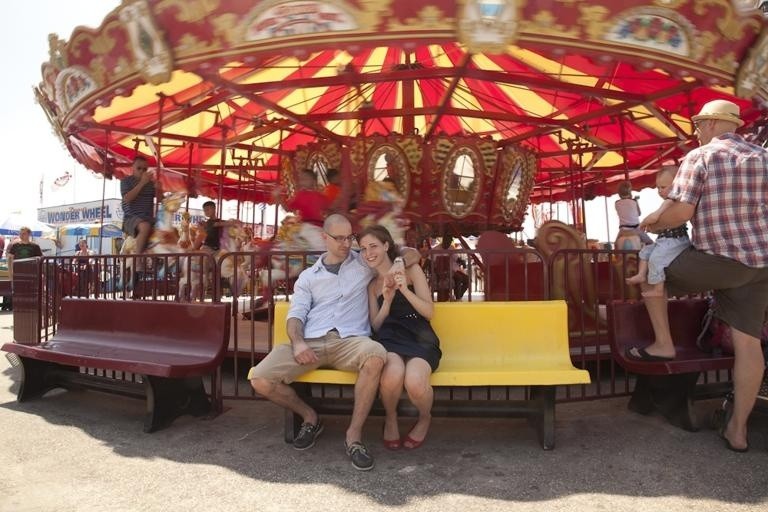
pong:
[718,426,749,452]
[625,343,675,361]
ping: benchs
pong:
[607,296,734,431]
[1,296,231,433]
[274,300,590,452]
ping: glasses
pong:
[325,232,358,243]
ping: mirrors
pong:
[278,131,536,240]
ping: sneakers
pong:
[344,440,375,470]
[293,412,324,451]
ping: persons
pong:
[72,240,97,272]
[615,180,654,245]
[356,225,442,451]
[120,156,155,255]
[200,201,233,254]
[246,213,423,471]
[624,166,691,298]
[427,231,470,302]
[624,99,768,453]
[7,227,43,281]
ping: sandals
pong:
[403,416,431,449]
[383,420,401,449]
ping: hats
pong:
[691,100,745,128]
